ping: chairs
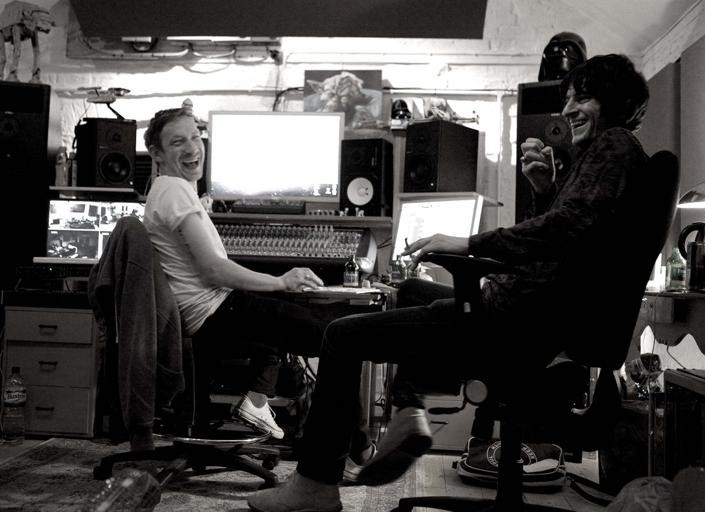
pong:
[91,216,281,509]
[390,144,681,511]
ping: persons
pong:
[141,109,381,486]
[247,53,650,512]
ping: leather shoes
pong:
[244,468,347,512]
[357,406,433,486]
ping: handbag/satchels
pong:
[458,436,566,494]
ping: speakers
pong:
[514,79,574,225]
[75,118,137,200]
[403,120,480,192]
[341,137,394,218]
[0,80,52,289]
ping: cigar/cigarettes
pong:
[404,237,412,259]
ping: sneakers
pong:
[233,396,284,441]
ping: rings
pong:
[304,274,310,279]
[520,156,526,163]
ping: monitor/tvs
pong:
[206,111,346,205]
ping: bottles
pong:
[2,366,28,446]
[343,254,359,288]
[54,146,68,184]
[665,248,688,292]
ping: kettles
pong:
[678,219,705,291]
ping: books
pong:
[302,284,382,295]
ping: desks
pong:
[1,282,383,440]
